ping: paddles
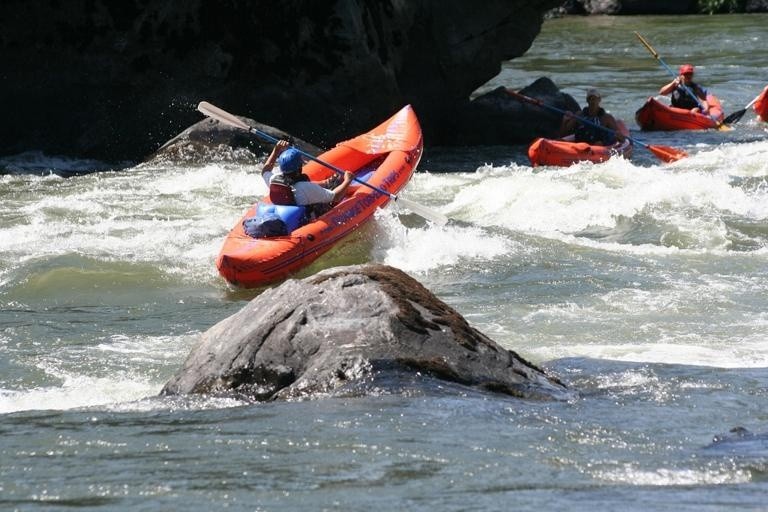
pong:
[198,100,449,228]
[633,30,735,131]
[498,87,688,164]
[722,94,760,126]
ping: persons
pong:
[560,89,625,147]
[262,139,355,219]
[659,63,709,114]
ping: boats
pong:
[528,118,632,166]
[636,95,723,131]
[752,85,768,123]
[215,103,424,290]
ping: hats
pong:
[587,89,600,100]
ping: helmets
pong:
[680,64,694,75]
[279,149,302,173]
